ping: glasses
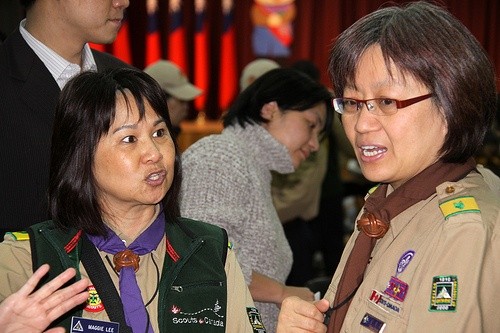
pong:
[330,91,436,118]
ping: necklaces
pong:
[0,68,267,333]
[102,250,159,333]
[318,277,364,324]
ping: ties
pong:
[82,205,168,333]
[325,155,478,332]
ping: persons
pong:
[177,67,334,333]
[143,61,202,125]
[0,0,141,241]
[163,84,166,85]
[276,2,500,333]
[240,59,356,287]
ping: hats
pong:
[240,59,282,92]
[143,59,201,102]
